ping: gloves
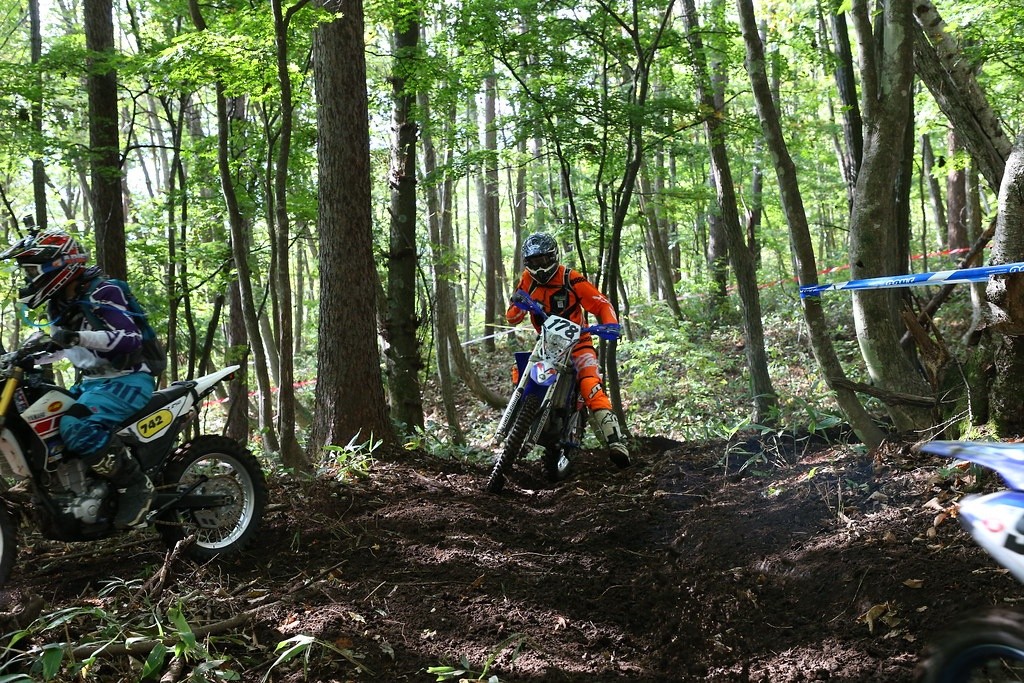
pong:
[46,330,79,353]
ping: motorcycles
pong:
[483,289,624,494]
[0,329,270,588]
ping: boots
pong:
[594,409,632,470]
[83,434,159,529]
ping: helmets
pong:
[520,234,559,285]
[0,232,88,309]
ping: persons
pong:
[507,231,629,468]
[0,228,158,528]
